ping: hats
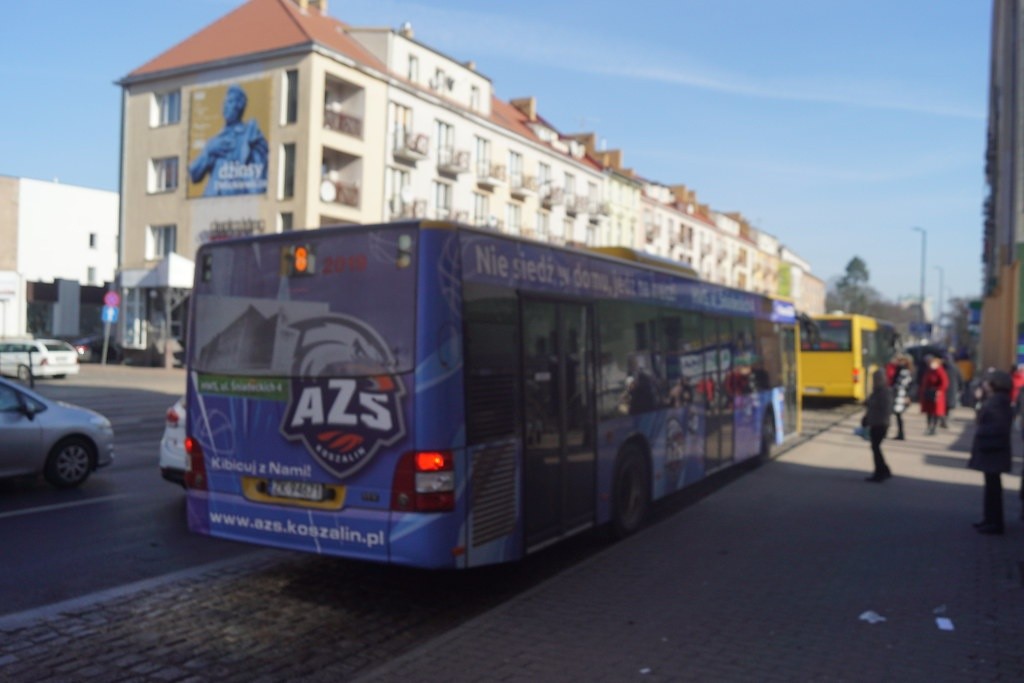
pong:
[984,371,1013,390]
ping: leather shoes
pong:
[976,521,1004,533]
[973,518,990,527]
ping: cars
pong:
[0,375,115,490]
[0,338,80,380]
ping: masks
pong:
[929,362,938,370]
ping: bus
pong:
[182,218,822,601]
[796,311,903,404]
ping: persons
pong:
[888,351,958,440]
[189,86,267,195]
[626,359,777,480]
[1007,366,1024,422]
[862,371,892,485]
[968,372,1012,535]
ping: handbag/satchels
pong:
[860,414,867,426]
[923,386,937,403]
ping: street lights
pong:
[912,226,926,341]
